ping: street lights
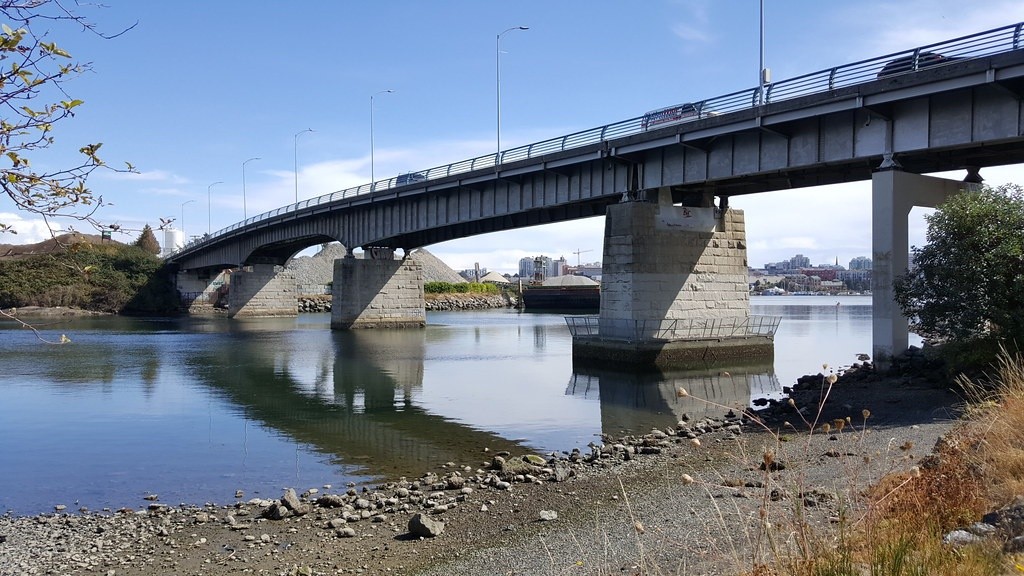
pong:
[242,157,262,220]
[294,127,312,204]
[208,181,225,235]
[370,89,396,184]
[497,25,531,165]
[181,199,195,232]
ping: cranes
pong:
[572,249,594,267]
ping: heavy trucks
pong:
[641,101,721,132]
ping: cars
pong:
[876,52,965,80]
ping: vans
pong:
[396,173,427,188]
